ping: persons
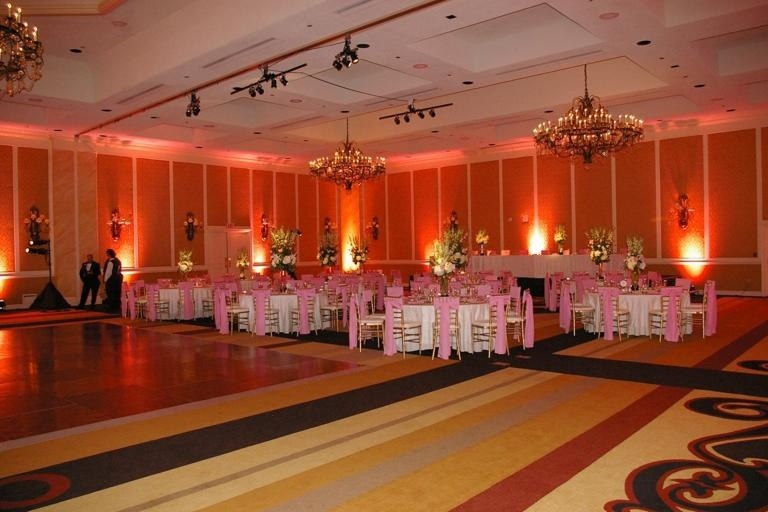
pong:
[76,249,123,310]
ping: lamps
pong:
[0,0,48,100]
[182,88,204,117]
[225,55,309,101]
[531,56,653,170]
[394,109,436,127]
[309,109,394,192]
[330,26,366,77]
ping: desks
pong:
[583,289,689,336]
[394,300,498,353]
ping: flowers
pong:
[182,216,198,227]
[624,233,647,271]
[427,227,469,291]
[24,211,51,227]
[178,248,194,276]
[475,230,489,244]
[585,224,616,263]
[269,224,299,278]
[347,232,368,264]
[105,213,130,228]
[553,224,568,243]
[235,249,250,269]
[316,240,338,267]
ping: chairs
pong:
[128,267,386,336]
[386,265,664,312]
[507,291,532,351]
[682,281,718,338]
[350,294,385,353]
[383,297,422,359]
[650,287,684,342]
[471,296,511,358]
[559,283,595,337]
[674,279,692,290]
[432,296,461,359]
[596,288,629,342]
